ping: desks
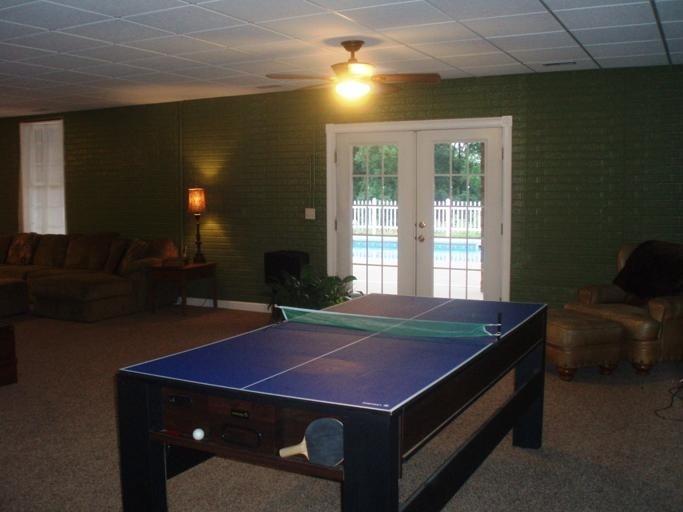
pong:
[115,292,548,512]
[264,250,308,316]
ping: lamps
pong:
[329,41,376,108]
[188,189,207,264]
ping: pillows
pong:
[117,237,153,275]
[32,234,69,266]
[66,237,101,271]
[4,232,38,264]
[110,238,126,263]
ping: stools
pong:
[545,308,624,381]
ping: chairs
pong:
[563,243,682,375]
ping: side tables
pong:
[149,259,220,313]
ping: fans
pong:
[266,40,441,95]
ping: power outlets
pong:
[305,207,315,220]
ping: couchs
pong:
[0,233,177,322]
